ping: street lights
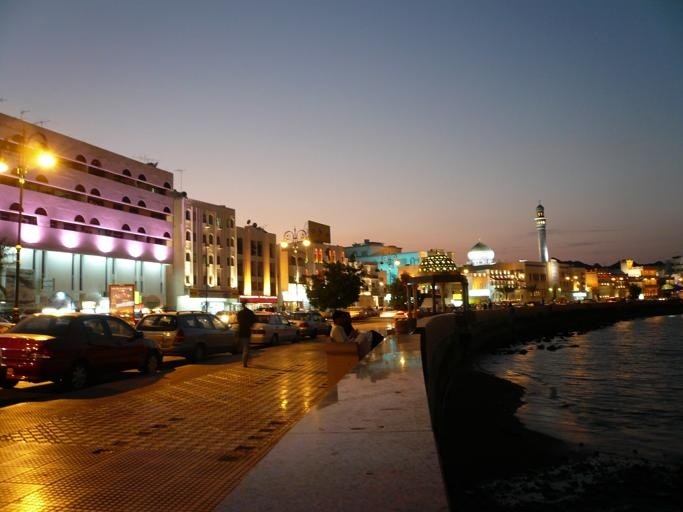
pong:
[0,130,56,327]
[280,227,310,312]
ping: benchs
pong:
[325,336,360,391]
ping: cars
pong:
[281,312,331,341]
[216,310,239,326]
[133,311,243,363]
[0,312,164,393]
[344,306,377,320]
[229,311,299,348]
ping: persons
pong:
[327,310,385,358]
[235,297,256,368]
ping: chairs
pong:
[67,320,88,339]
[160,321,167,327]
